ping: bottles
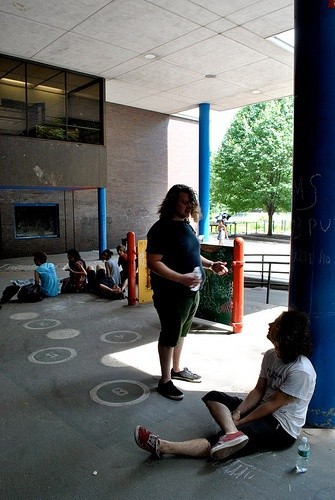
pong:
[296,437,311,473]
[190,266,203,291]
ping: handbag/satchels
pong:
[17,284,43,302]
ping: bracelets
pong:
[232,410,242,414]
[209,262,214,270]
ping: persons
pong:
[34,244,139,300]
[134,311,317,460]
[146,184,229,400]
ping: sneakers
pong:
[171,367,203,383]
[135,426,163,458]
[210,431,249,459]
[158,379,184,400]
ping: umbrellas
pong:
[215,212,231,220]
